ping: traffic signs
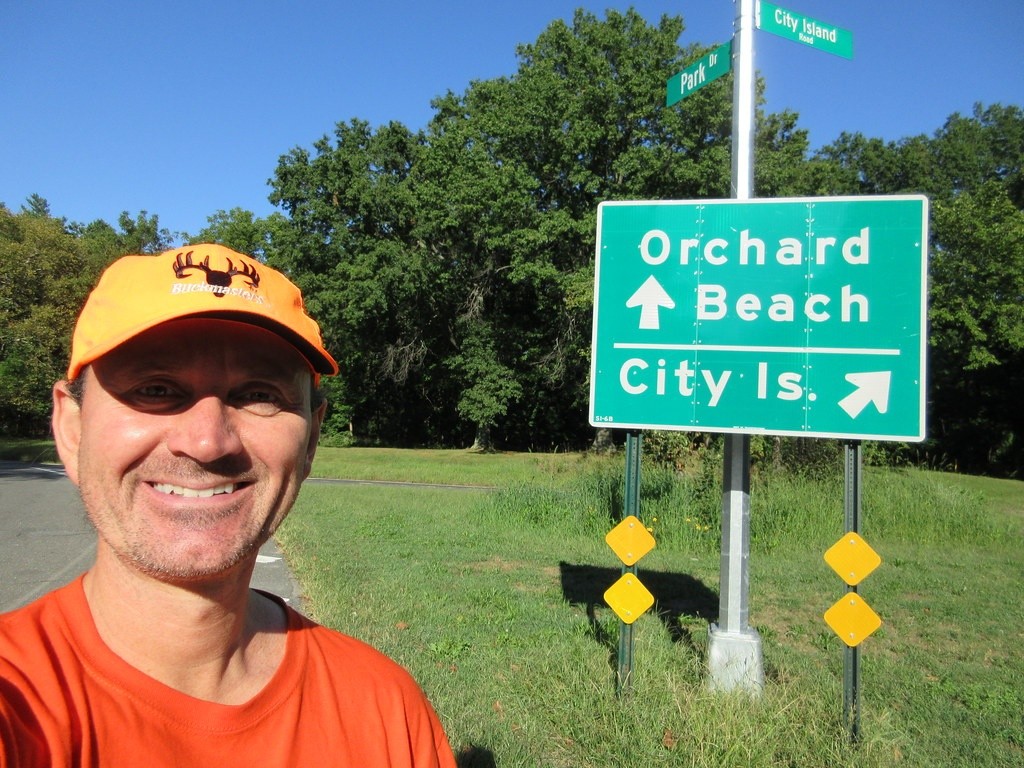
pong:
[589,195,929,442]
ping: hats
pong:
[67,243,339,392]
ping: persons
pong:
[1,243,457,768]
